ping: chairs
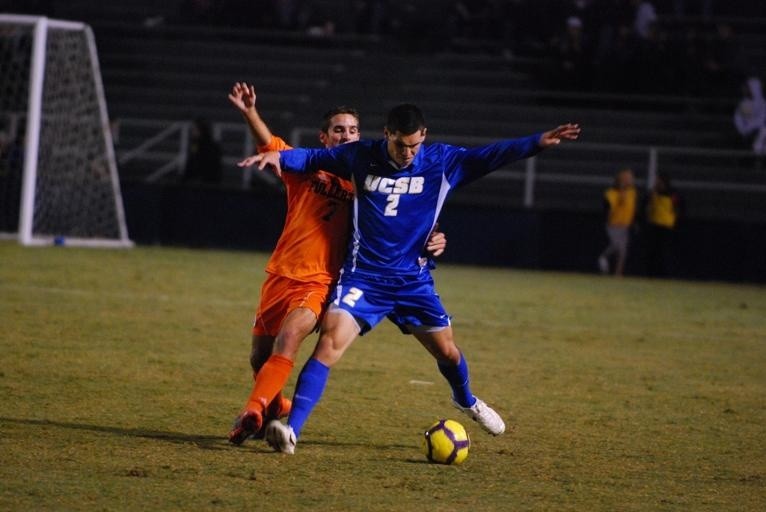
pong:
[225,395,297,455]
[448,393,506,436]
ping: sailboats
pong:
[422,419,471,464]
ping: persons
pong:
[644,165,678,230]
[225,76,448,445]
[732,75,766,154]
[593,165,638,274]
[233,98,582,457]
